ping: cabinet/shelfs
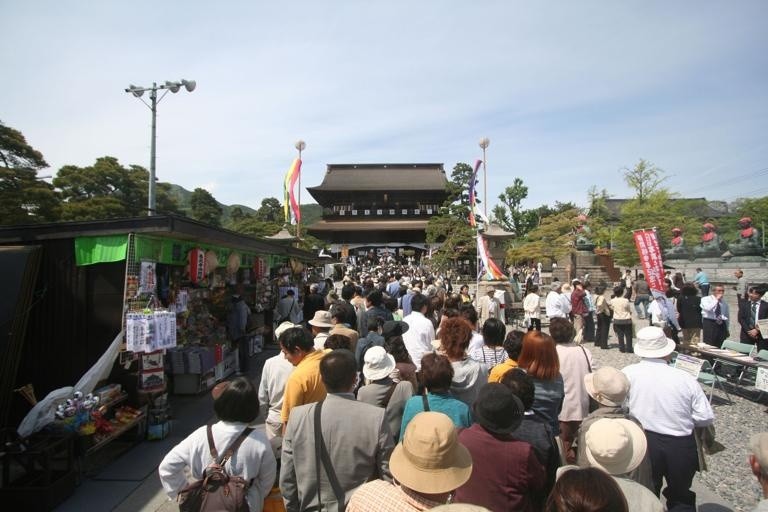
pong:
[160,270,314,396]
[0,381,172,471]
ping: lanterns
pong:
[187,251,205,283]
[227,254,240,273]
[255,257,266,279]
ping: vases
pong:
[574,245,600,251]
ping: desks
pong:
[678,342,768,391]
[505,303,551,330]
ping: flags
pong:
[634,231,667,300]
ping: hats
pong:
[470,382,524,434]
[362,345,396,380]
[486,286,496,292]
[634,326,676,358]
[383,320,409,337]
[308,309,335,327]
[389,411,473,495]
[585,418,648,474]
[274,321,303,340]
[584,366,631,407]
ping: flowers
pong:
[574,214,600,244]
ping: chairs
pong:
[674,353,732,406]
[668,351,678,367]
[709,340,758,385]
[733,349,767,402]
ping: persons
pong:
[304,252,716,512]
[157,290,336,512]
[748,434,768,512]
[622,269,768,378]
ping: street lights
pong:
[294,139,307,247]
[476,137,492,220]
[126,78,196,217]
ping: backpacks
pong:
[177,423,255,512]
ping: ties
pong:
[715,303,723,325]
[750,303,757,327]
